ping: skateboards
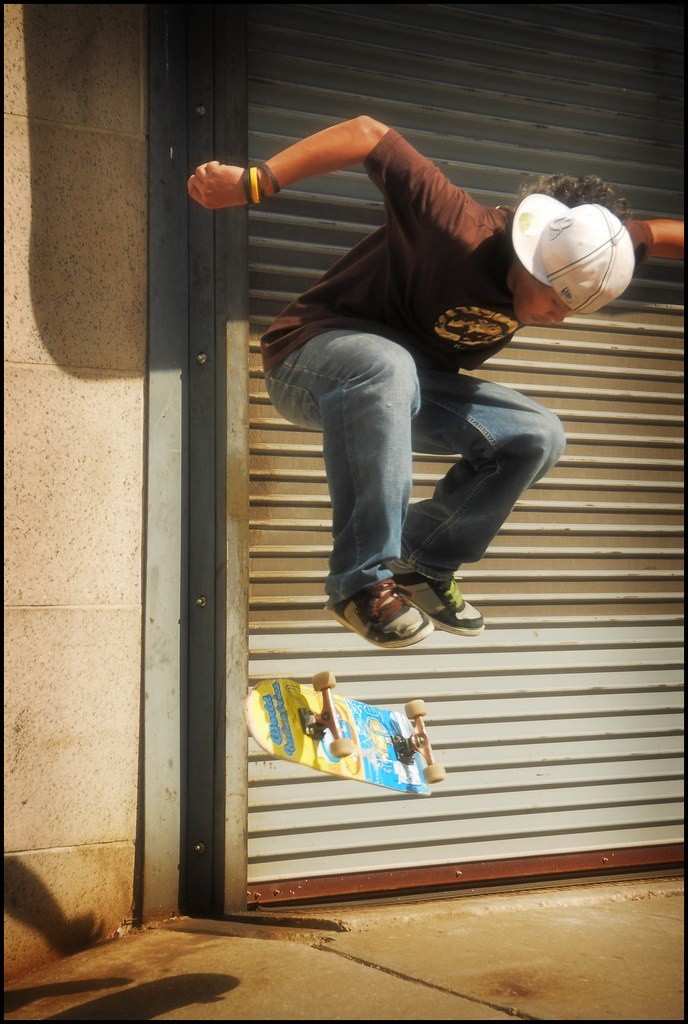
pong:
[242,673,449,796]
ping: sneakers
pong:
[323,561,485,648]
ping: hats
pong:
[512,194,635,315]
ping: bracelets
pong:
[243,163,281,206]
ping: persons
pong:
[186,114,685,649]
